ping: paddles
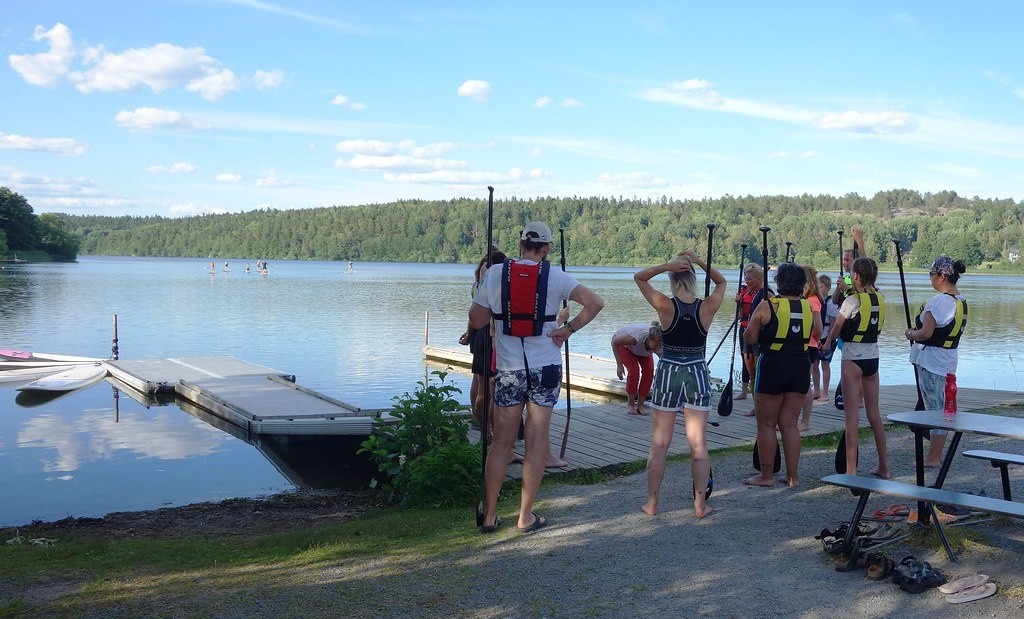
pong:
[558,228,571,458]
[751,228,782,475]
[706,224,715,298]
[893,238,931,441]
[785,241,796,262]
[480,186,495,523]
[834,240,860,475]
[836,229,850,411]
[717,245,749,416]
[707,318,735,366]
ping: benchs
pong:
[961,449,1024,501]
[818,470,1024,563]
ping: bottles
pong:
[944,373,957,415]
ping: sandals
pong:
[891,556,931,584]
[814,528,844,553]
[899,569,949,594]
[836,525,872,546]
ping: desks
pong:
[888,410,1024,531]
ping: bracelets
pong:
[568,322,576,332]
[908,331,912,340]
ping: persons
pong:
[812,275,839,405]
[224,261,228,267]
[634,250,727,518]
[832,249,859,308]
[210,262,214,269]
[246,263,250,268]
[458,251,569,468]
[776,265,823,431]
[470,220,604,532]
[349,260,353,270]
[612,320,663,416]
[257,260,260,270]
[261,261,267,270]
[905,257,968,468]
[830,224,891,479]
[732,263,775,417]
[743,263,815,487]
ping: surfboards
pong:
[13,363,108,391]
[0,349,113,362]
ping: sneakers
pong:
[977,490,987,497]
[960,491,986,516]
[907,506,950,526]
[936,503,971,519]
[869,523,901,543]
[930,504,957,522]
[837,519,878,535]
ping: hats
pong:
[520,220,553,242]
[930,256,956,275]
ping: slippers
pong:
[939,573,989,594]
[515,511,548,533]
[946,583,997,604]
[866,553,896,579]
[878,503,910,516]
[481,514,503,532]
[836,548,873,572]
[861,508,905,522]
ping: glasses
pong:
[928,270,939,277]
